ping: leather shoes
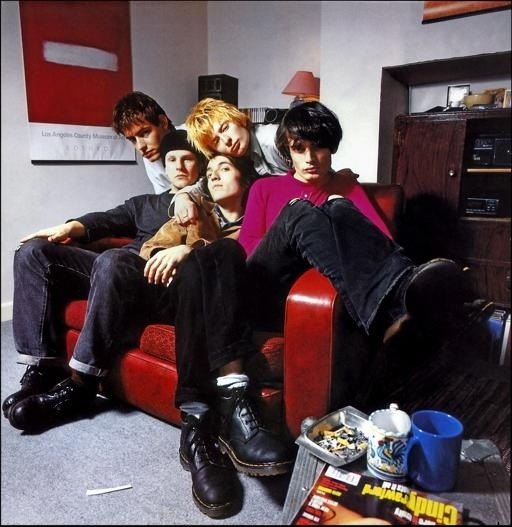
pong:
[378,258,497,369]
[180,410,242,519]
[2,365,97,432]
[214,388,295,476]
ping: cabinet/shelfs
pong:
[392,112,511,311]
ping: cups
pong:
[367,403,464,493]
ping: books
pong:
[290,463,464,526]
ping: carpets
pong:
[361,362,511,479]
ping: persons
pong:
[239,101,494,400]
[113,93,186,193]
[186,98,293,177]
[5,130,203,430]
[141,154,294,517]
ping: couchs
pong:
[61,184,404,441]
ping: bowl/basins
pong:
[463,95,494,110]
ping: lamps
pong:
[280,70,320,112]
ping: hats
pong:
[159,129,202,167]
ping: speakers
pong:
[198,74,238,109]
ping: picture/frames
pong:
[446,83,471,109]
